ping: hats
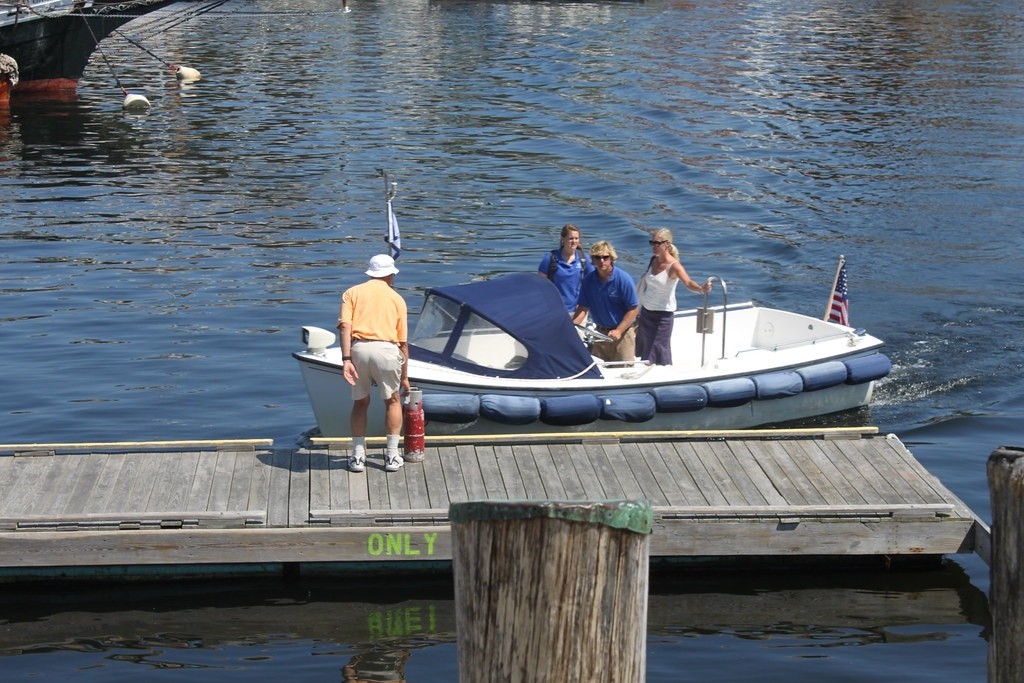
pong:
[364,254,399,278]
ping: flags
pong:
[828,257,850,326]
[383,200,401,260]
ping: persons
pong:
[336,253,411,472]
[538,224,595,341]
[571,241,639,367]
[634,228,712,366]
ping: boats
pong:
[290,268,891,444]
[0,0,184,116]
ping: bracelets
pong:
[342,356,351,360]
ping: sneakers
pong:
[347,455,365,472]
[385,455,403,471]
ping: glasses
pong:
[649,239,667,247]
[592,255,610,261]
